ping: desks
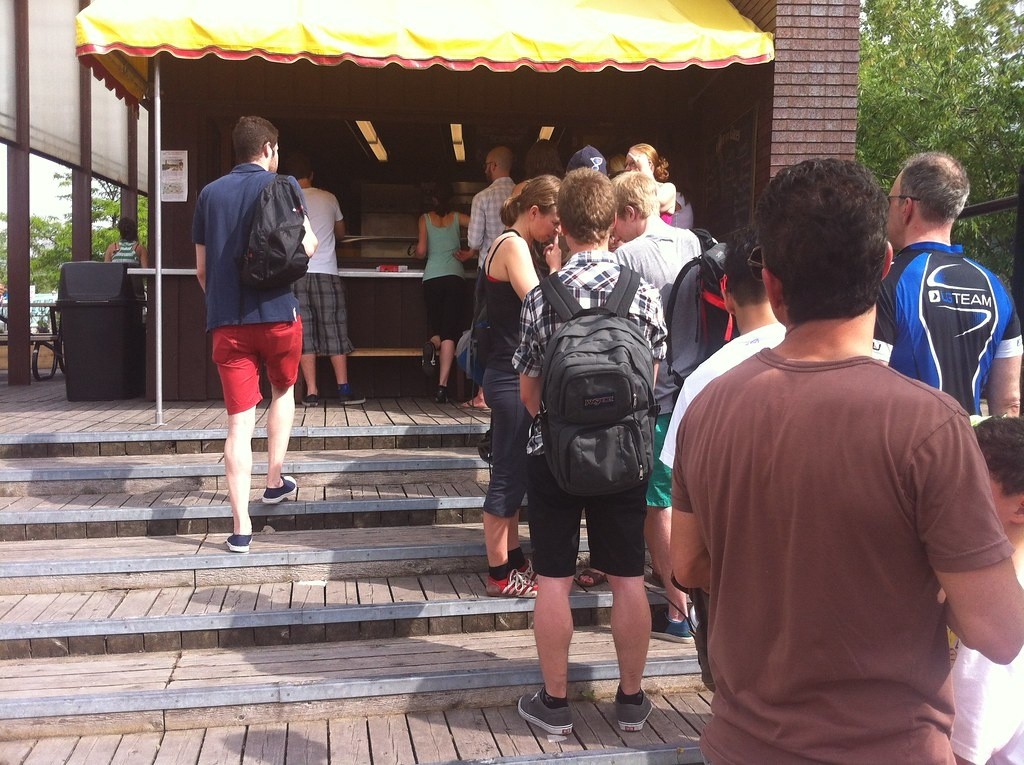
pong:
[0,302,65,381]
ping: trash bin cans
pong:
[55,261,148,402]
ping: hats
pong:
[566,145,607,177]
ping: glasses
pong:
[482,162,492,171]
[747,246,768,284]
[624,159,636,169]
[877,194,919,210]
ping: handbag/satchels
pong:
[241,175,309,292]
[455,326,484,384]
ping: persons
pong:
[282,151,366,406]
[192,114,305,553]
[105,217,148,268]
[422,141,1024,765]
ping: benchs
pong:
[0,331,63,343]
[295,348,464,402]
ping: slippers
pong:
[574,568,607,588]
[459,398,490,412]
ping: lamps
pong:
[355,121,388,161]
[536,125,554,143]
[450,123,466,162]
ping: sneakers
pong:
[300,394,318,408]
[486,558,539,597]
[421,342,437,371]
[226,535,253,553]
[518,688,573,736]
[262,476,297,504]
[614,692,653,732]
[648,606,694,644]
[433,386,448,403]
[340,391,368,405]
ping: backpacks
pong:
[538,265,660,497]
[664,229,740,386]
